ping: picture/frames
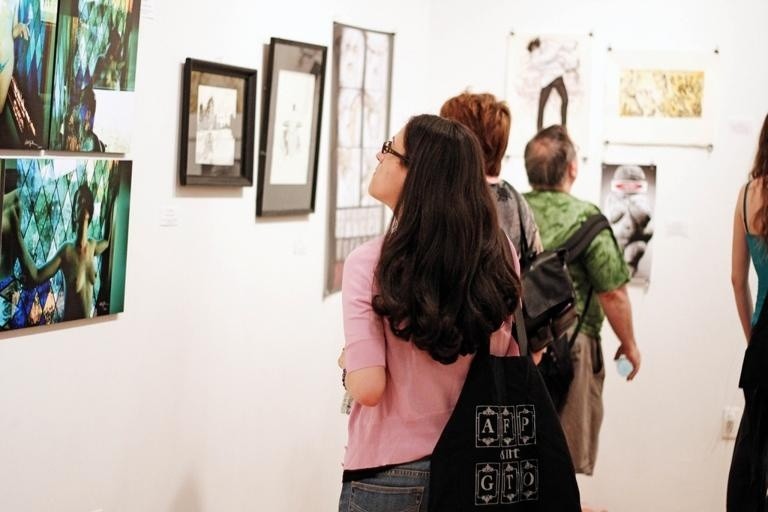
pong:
[181,60,256,188]
[259,37,327,217]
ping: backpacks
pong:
[536,214,621,420]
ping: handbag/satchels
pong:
[425,230,583,512]
[502,181,578,353]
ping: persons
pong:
[10,185,110,321]
[0,0,30,116]
[338,114,583,512]
[520,125,641,475]
[726,115,768,512]
[439,93,578,367]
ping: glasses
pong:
[382,140,410,164]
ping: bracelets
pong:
[342,369,347,391]
